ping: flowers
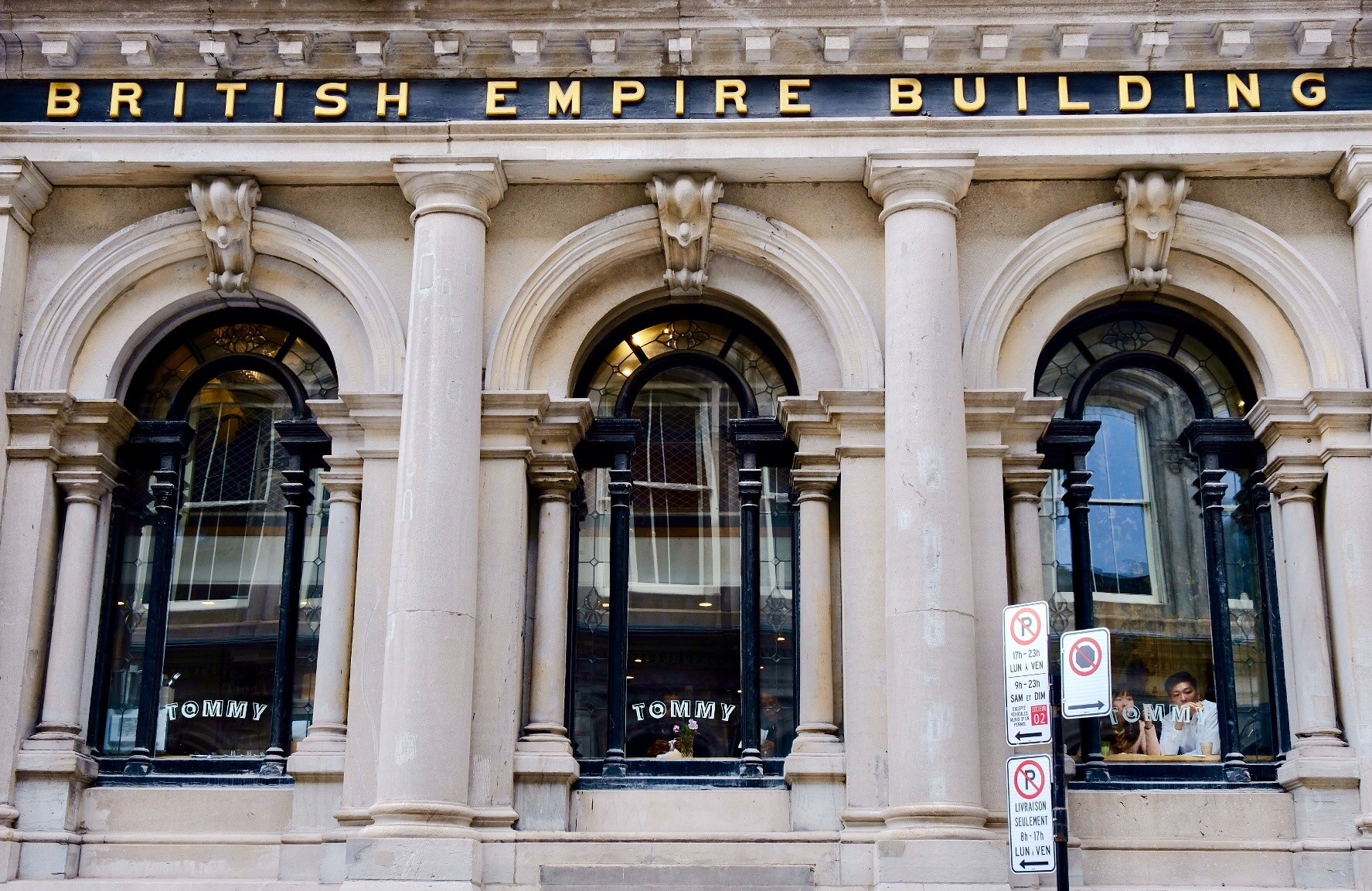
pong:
[670,719,699,732]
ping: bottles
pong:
[1064,744,1076,780]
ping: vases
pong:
[677,730,694,759]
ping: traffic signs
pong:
[1005,753,1058,875]
[999,600,1052,748]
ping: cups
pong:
[1200,742,1213,755]
[1101,741,1110,757]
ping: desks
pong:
[1098,752,1274,764]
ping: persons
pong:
[1078,683,1160,765]
[632,683,714,759]
[1118,658,1167,739]
[744,686,796,761]
[1156,671,1225,758]
